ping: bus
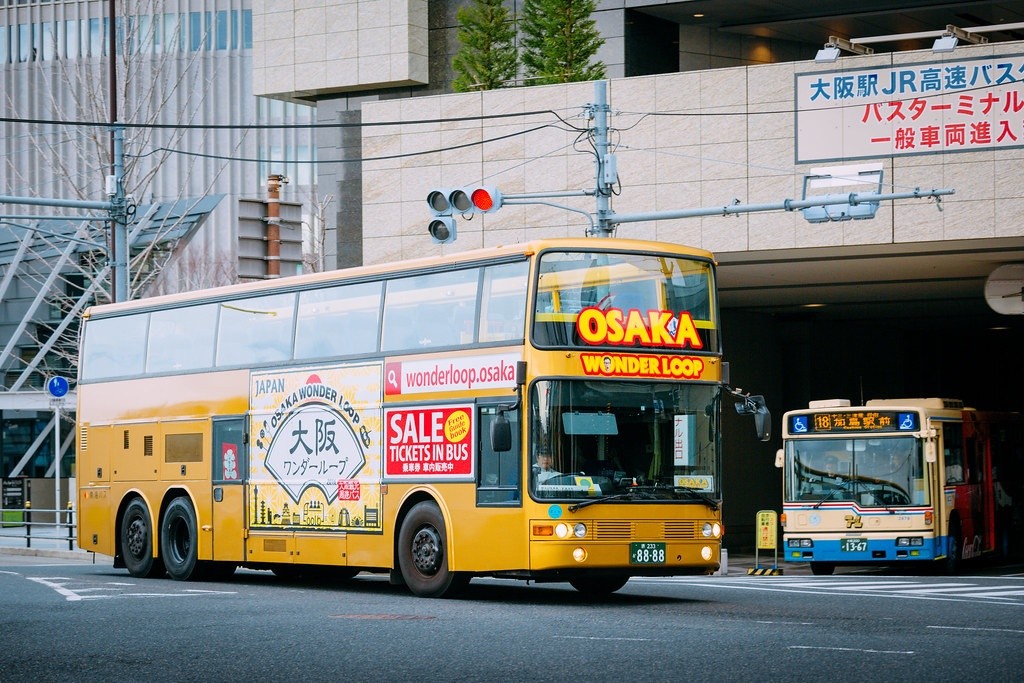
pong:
[75,238,772,597]
[775,396,1024,579]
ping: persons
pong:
[534,447,562,492]
[604,357,612,371]
[809,456,852,490]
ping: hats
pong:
[825,456,838,463]
[536,444,556,455]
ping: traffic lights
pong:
[427,187,501,243]
